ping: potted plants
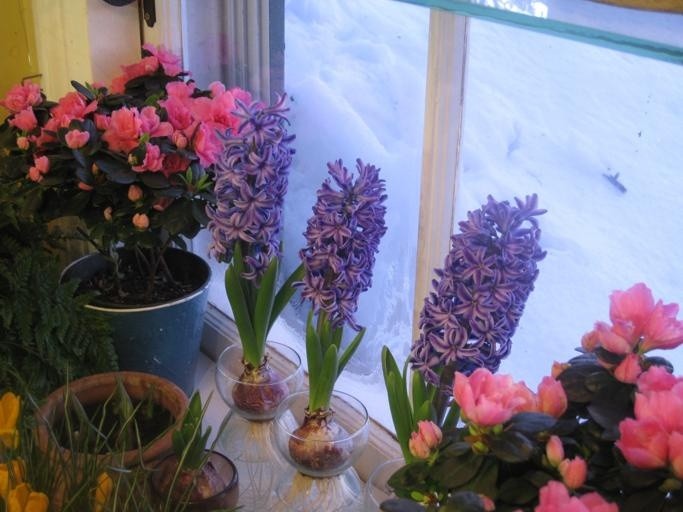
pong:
[151,392,240,511]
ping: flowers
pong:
[0,36,273,292]
[278,151,390,473]
[408,280,682,511]
[198,93,311,422]
[0,362,233,512]
[375,188,550,498]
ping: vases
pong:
[58,246,212,380]
[31,370,196,509]
[210,338,304,511]
[258,389,373,511]
[356,453,482,510]
